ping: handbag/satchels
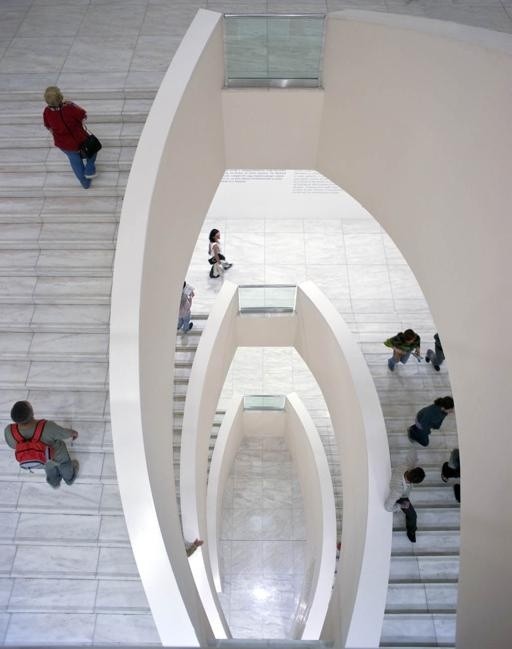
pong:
[79,133,103,159]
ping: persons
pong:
[425,332,446,372]
[43,86,99,190]
[406,395,455,448]
[3,401,81,490]
[184,538,204,558]
[175,279,193,333]
[441,449,461,504]
[208,228,232,279]
[383,463,426,544]
[383,328,422,371]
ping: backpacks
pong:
[10,419,56,472]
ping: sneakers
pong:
[84,170,98,190]
[52,458,80,490]
